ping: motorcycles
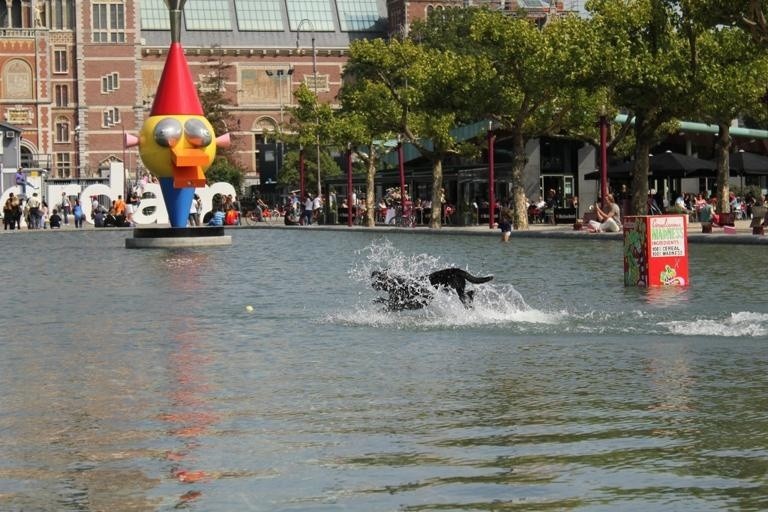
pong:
[282,203,308,225]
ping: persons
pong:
[498,208,512,242]
[16,167,39,193]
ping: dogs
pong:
[370,267,494,314]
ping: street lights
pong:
[347,140,353,228]
[265,69,295,202]
[296,18,321,196]
[487,119,496,229]
[300,139,304,226]
[397,134,409,227]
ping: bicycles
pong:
[389,201,418,226]
[246,204,280,226]
